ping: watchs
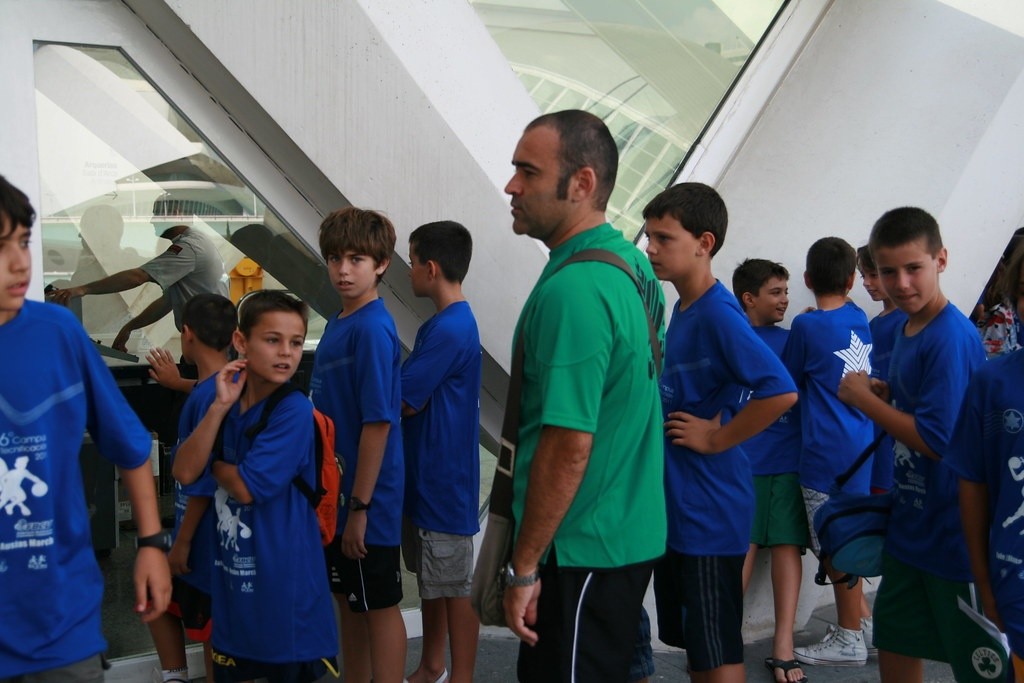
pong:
[500,558,541,585]
[347,496,371,511]
[135,527,173,555]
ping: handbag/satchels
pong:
[468,511,514,627]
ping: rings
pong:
[63,295,67,297]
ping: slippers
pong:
[764,657,809,683]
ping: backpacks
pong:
[258,382,342,549]
[810,429,895,589]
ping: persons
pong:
[837,208,987,683]
[169,289,344,683]
[643,183,799,683]
[310,208,409,683]
[43,204,150,330]
[941,238,1024,683]
[47,189,238,380]
[783,234,880,671]
[497,106,667,683]
[389,219,480,683]
[144,296,245,683]
[721,258,811,683]
[0,175,174,683]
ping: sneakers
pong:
[826,614,879,662]
[793,624,869,667]
